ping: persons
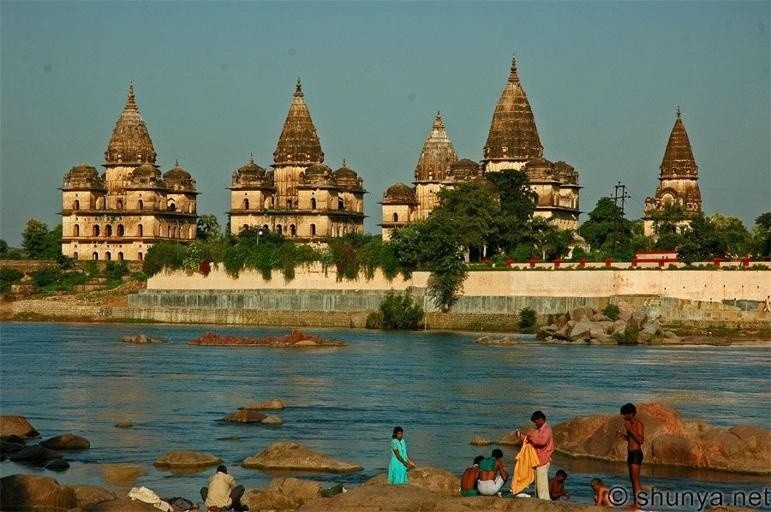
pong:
[591,478,616,509]
[200,464,245,511]
[549,470,569,501]
[616,403,644,511]
[387,426,416,485]
[476,448,509,498]
[459,454,481,497]
[519,411,554,501]
